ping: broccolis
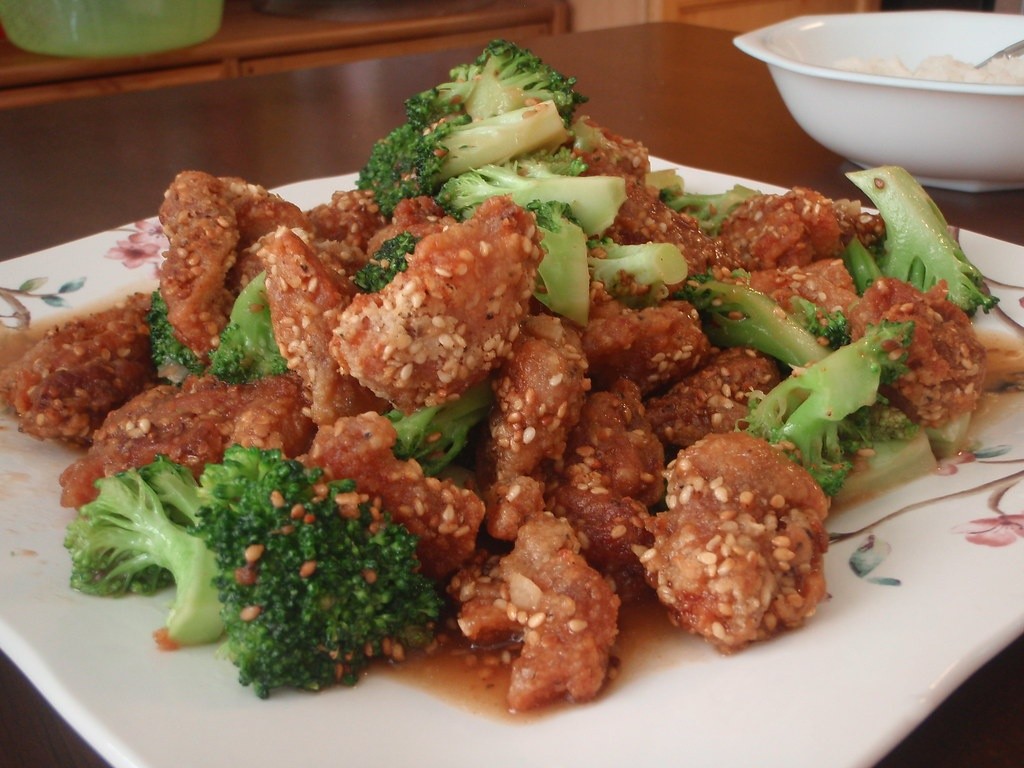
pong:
[61,42,1004,697]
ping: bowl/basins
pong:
[734,10,1024,191]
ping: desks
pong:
[0,20,1024,768]
[0,0,573,112]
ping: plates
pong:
[0,154,1024,768]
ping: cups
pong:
[0,1,223,55]
